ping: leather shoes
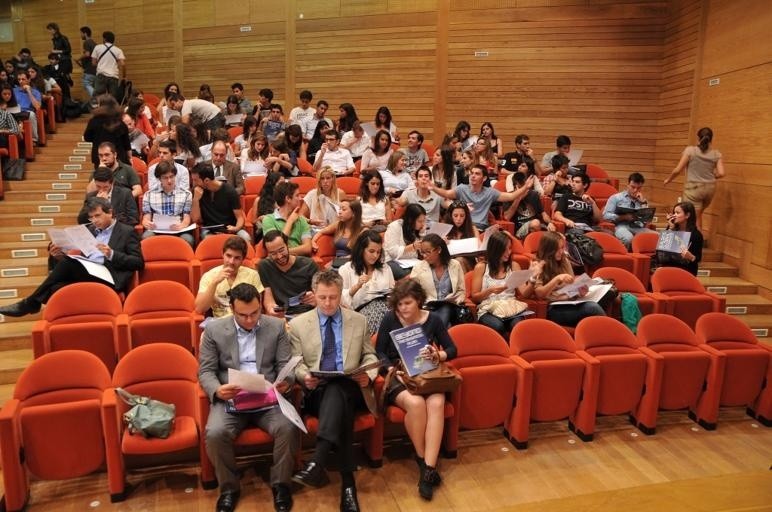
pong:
[417,456,441,500]
[0,299,40,317]
[272,482,291,511]
[216,486,240,512]
[340,484,359,512]
[291,459,322,489]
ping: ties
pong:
[319,315,338,371]
[214,166,221,177]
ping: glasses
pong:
[88,214,103,222]
[420,247,436,255]
[325,138,336,141]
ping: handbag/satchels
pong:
[483,298,528,318]
[602,278,618,299]
[566,231,595,258]
[123,396,176,439]
[398,356,463,394]
[3,158,26,180]
[452,305,472,325]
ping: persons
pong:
[375,278,457,501]
[0,47,74,202]
[0,197,145,318]
[81,81,725,338]
[0,22,126,82]
[197,282,296,511]
[287,267,381,511]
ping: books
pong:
[307,359,386,383]
[389,321,439,376]
